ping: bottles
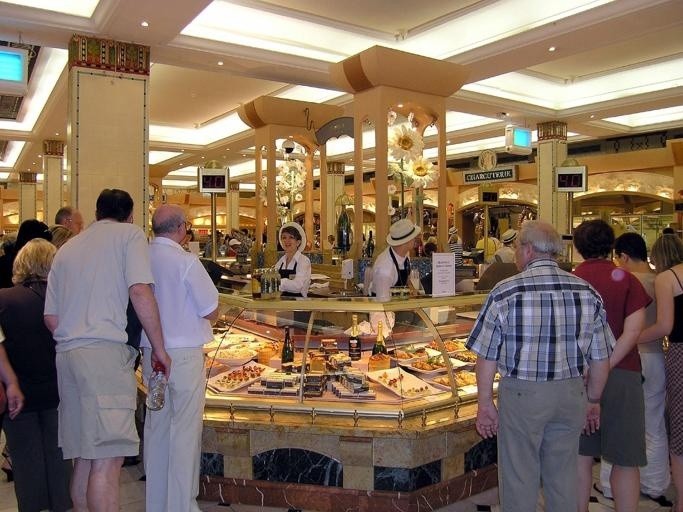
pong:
[371,321,388,357]
[281,325,292,373]
[337,205,350,252]
[366,231,373,257]
[250,267,280,300]
[144,362,167,411]
[349,315,361,361]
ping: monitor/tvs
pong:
[420,272,432,294]
[199,257,235,278]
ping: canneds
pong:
[400,287,411,300]
[390,286,400,300]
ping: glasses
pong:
[178,221,192,231]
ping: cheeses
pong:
[368,352,390,373]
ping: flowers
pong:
[403,155,441,227]
[388,124,424,219]
[253,155,306,226]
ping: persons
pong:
[0,216,55,482]
[46,223,76,248]
[0,235,77,512]
[183,228,258,259]
[314,231,336,248]
[459,220,619,512]
[0,324,28,422]
[42,187,171,511]
[182,223,527,263]
[120,294,145,468]
[561,216,656,512]
[264,221,313,325]
[415,223,522,263]
[593,230,671,501]
[135,203,221,512]
[52,205,84,236]
[631,232,682,512]
[367,218,422,333]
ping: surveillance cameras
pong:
[282,140,295,155]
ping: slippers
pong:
[593,482,616,501]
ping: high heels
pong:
[1,457,15,482]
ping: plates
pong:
[202,333,277,392]
[364,366,443,399]
[383,338,479,391]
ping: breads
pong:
[394,339,501,388]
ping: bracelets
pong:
[587,393,600,405]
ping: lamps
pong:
[331,189,355,234]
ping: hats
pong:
[449,226,458,235]
[228,238,242,246]
[278,222,307,252]
[500,228,519,244]
[386,219,421,246]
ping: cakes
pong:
[204,320,375,402]
[378,373,429,399]
